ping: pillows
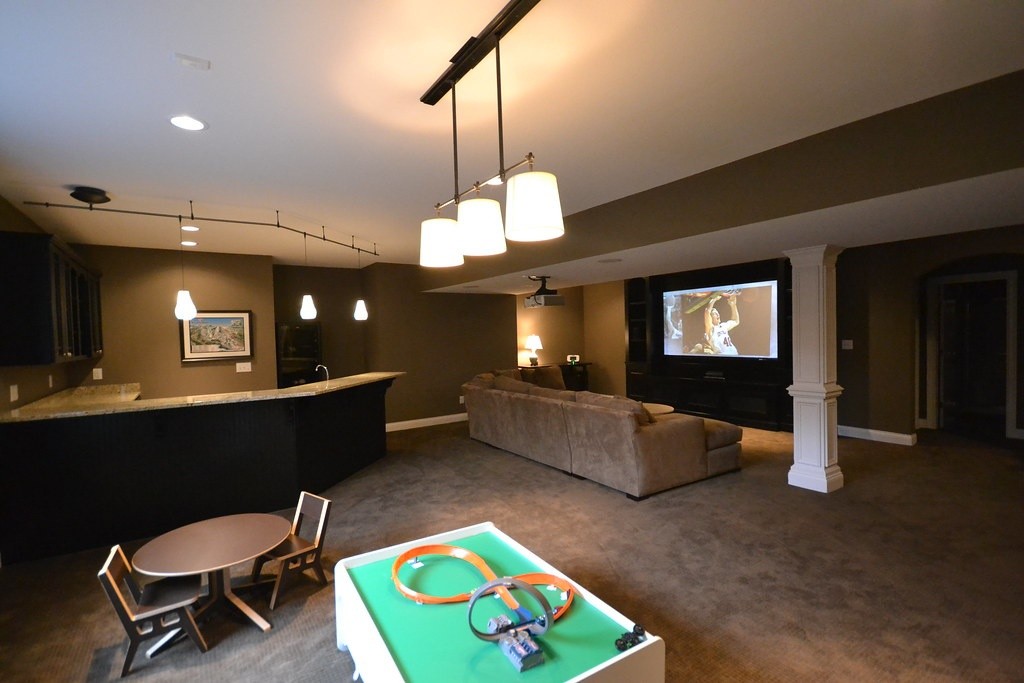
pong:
[534,366,566,390]
[613,394,658,424]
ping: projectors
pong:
[525,294,566,308]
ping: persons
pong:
[703,295,740,355]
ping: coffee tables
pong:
[642,402,674,415]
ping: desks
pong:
[337,521,666,683]
[131,513,292,660]
[542,363,594,391]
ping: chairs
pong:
[98,544,207,678]
[250,491,332,611]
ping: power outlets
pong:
[93,368,102,380]
[235,362,252,374]
[49,375,52,388]
[10,385,18,402]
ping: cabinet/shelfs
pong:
[625,257,793,434]
[0,230,103,366]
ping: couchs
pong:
[460,365,743,501]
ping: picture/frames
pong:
[179,309,254,364]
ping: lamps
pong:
[354,249,368,321]
[525,334,543,366]
[420,0,565,268]
[300,233,317,321]
[174,215,197,320]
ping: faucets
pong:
[315,365,329,381]
